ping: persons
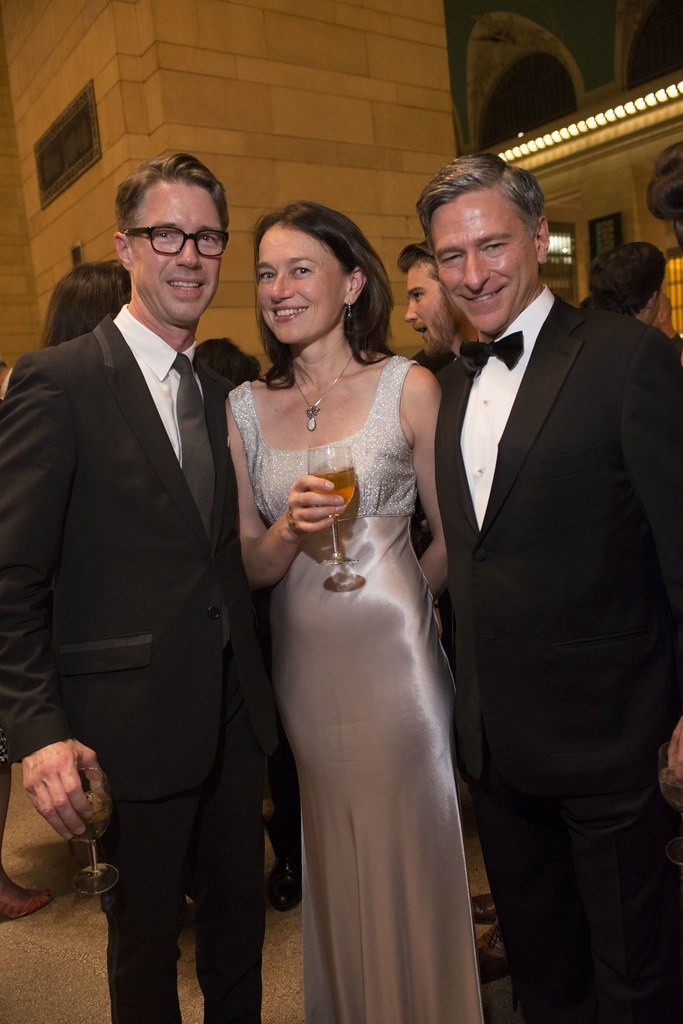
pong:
[0,152,683,1024]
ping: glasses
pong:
[122,227,229,257]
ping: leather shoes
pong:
[0,886,53,919]
[476,920,508,983]
[268,852,302,911]
[470,894,497,924]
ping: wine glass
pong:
[307,445,367,592]
[72,768,119,894]
[658,741,683,865]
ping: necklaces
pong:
[286,352,352,432]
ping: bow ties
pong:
[460,331,524,377]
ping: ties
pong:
[172,353,216,550]
